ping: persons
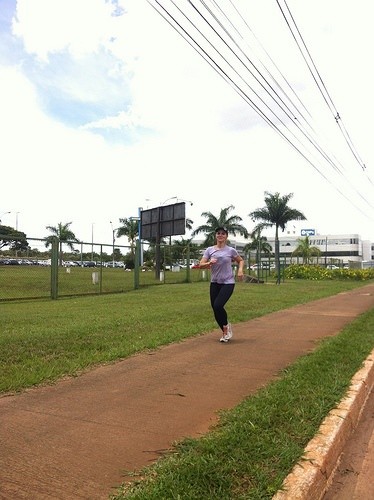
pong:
[198,226,243,342]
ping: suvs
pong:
[179,263,194,270]
[327,265,339,270]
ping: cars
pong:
[270,263,275,271]
[343,264,349,270]
[246,263,262,270]
[192,264,210,269]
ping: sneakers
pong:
[223,322,233,339]
[220,336,228,343]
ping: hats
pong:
[216,227,228,236]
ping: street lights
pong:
[0,258,126,269]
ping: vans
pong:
[262,263,269,269]
